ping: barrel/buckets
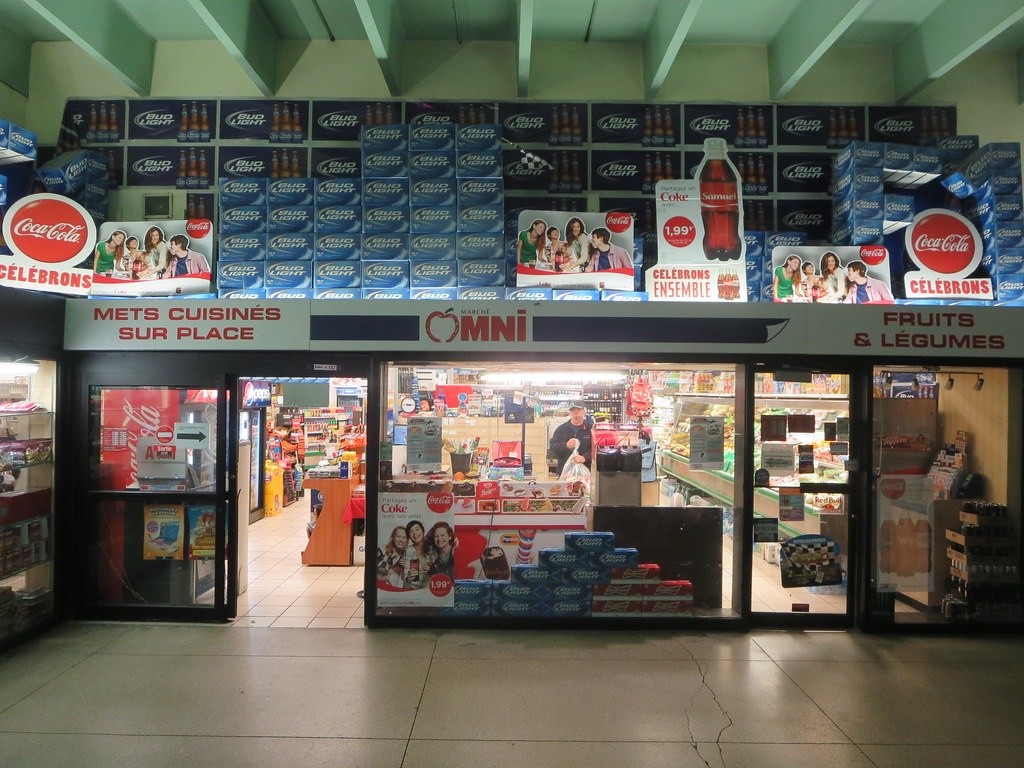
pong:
[450,452,471,476]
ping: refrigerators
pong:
[180,381,272,523]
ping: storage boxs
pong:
[947,547,1019,567]
[959,511,1008,527]
[642,601,695,617]
[540,584,593,600]
[610,564,661,584]
[589,548,639,568]
[945,528,1017,547]
[538,547,589,567]
[440,601,491,617]
[542,600,591,617]
[493,581,540,600]
[0,99,1024,306]
[353,535,365,566]
[0,438,52,472]
[452,581,492,600]
[561,567,609,585]
[339,461,351,479]
[563,531,615,552]
[511,564,561,585]
[949,566,1020,583]
[492,600,542,617]
[592,601,641,617]
[593,584,643,601]
[0,486,51,525]
[642,580,693,601]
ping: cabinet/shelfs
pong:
[0,411,53,642]
[300,367,849,575]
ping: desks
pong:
[875,475,987,613]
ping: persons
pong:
[549,400,592,482]
[517,216,633,272]
[385,520,454,590]
[93,226,210,280]
[773,251,893,304]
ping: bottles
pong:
[738,154,765,183]
[646,153,672,182]
[644,105,672,135]
[272,101,300,131]
[181,101,209,132]
[880,509,897,573]
[829,107,857,139]
[188,195,205,218]
[551,152,580,184]
[922,108,948,138]
[460,104,484,125]
[90,101,117,131]
[91,148,114,174]
[597,445,641,472]
[737,106,765,137]
[915,508,932,573]
[540,388,622,422]
[552,104,579,135]
[180,147,206,178]
[366,103,392,125]
[272,148,299,178]
[700,137,741,263]
[896,508,916,577]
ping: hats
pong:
[568,395,585,410]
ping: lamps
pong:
[974,374,984,391]
[944,373,954,391]
[882,372,892,391]
[912,373,921,391]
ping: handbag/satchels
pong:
[558,439,592,497]
